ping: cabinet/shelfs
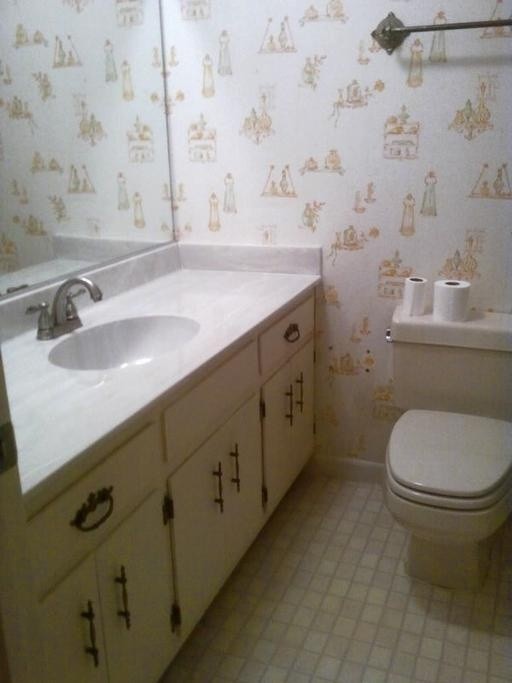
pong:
[29,294,314,682]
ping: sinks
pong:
[47,314,200,372]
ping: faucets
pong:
[51,276,103,327]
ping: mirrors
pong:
[1,0,174,300]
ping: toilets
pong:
[383,305,511,589]
